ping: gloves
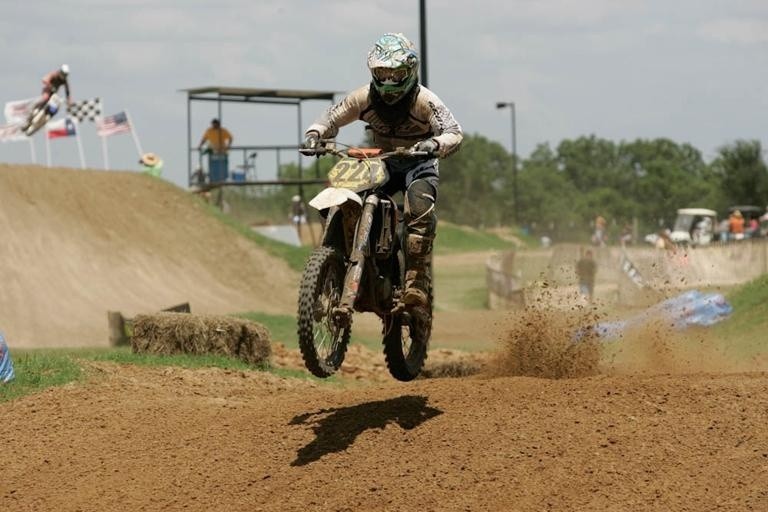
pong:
[297,133,323,157]
[410,138,438,158]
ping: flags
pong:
[0,95,136,141]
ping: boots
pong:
[399,230,435,308]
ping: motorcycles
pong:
[296,135,442,384]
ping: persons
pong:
[297,31,466,313]
[138,152,165,178]
[31,63,73,115]
[573,248,599,300]
[592,209,768,248]
[198,117,233,156]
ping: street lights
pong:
[496,101,518,226]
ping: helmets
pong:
[59,64,71,80]
[367,32,421,107]
